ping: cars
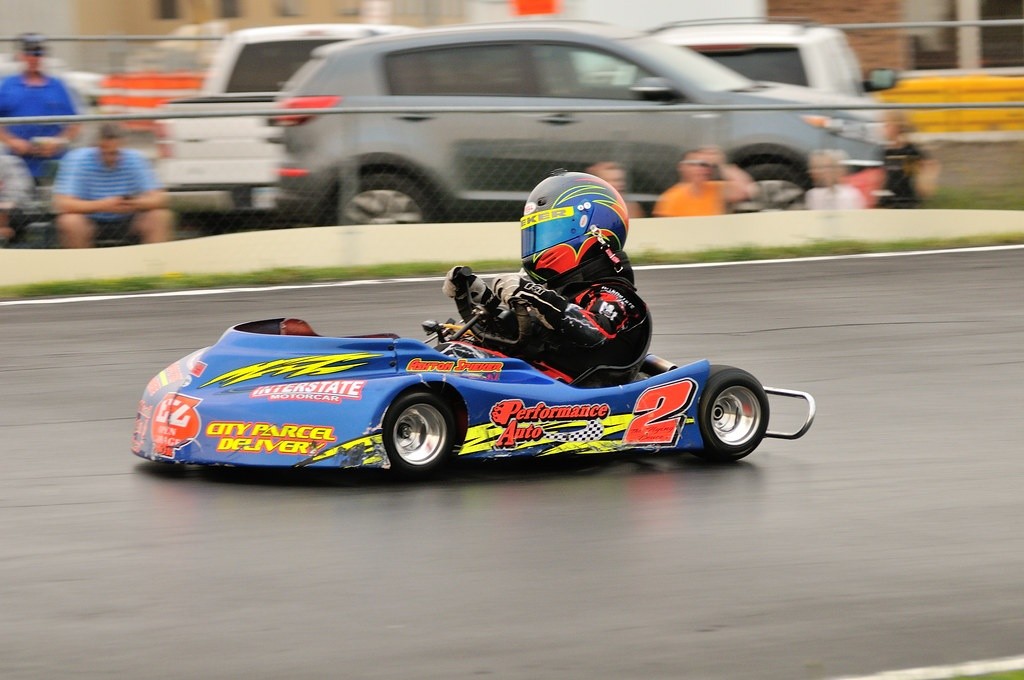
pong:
[265,23,888,227]
[573,28,895,102]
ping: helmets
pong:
[518,170,631,287]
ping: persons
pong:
[805,150,865,208]
[0,33,177,248]
[872,113,927,209]
[443,168,652,387]
[587,162,643,217]
[652,148,760,217]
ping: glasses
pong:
[21,45,45,58]
[680,159,718,170]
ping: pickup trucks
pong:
[151,24,430,215]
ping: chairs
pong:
[565,285,654,389]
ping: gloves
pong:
[508,280,568,319]
[443,266,496,306]
